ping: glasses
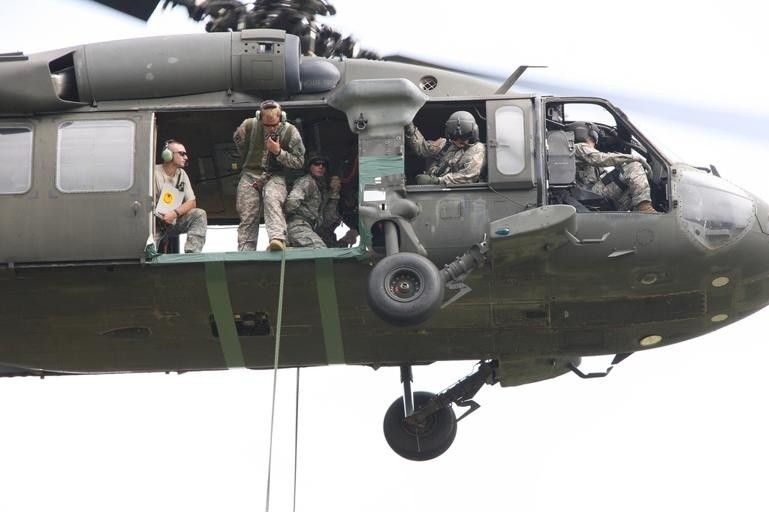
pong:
[263,122,281,127]
[174,150,188,157]
[313,160,329,168]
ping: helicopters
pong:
[0,0,769,461]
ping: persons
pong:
[155,138,208,254]
[284,149,342,249]
[405,111,487,184]
[565,121,657,213]
[232,100,307,251]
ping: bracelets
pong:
[175,209,180,217]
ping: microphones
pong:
[460,142,476,147]
[185,162,189,167]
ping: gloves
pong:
[404,119,416,138]
[415,173,440,185]
[639,157,655,183]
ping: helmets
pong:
[444,110,480,145]
[565,120,600,144]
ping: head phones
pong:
[255,103,288,124]
[160,139,175,162]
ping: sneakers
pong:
[269,237,286,252]
[638,200,661,216]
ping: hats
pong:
[259,98,280,112]
